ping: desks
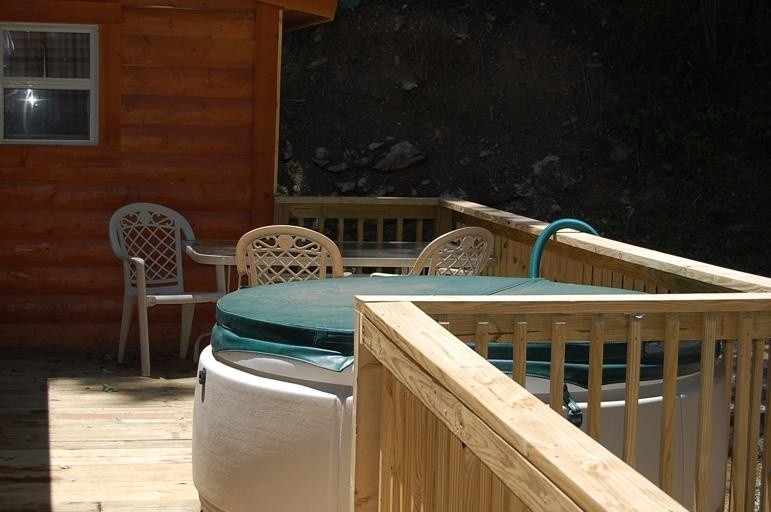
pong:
[178,235,496,365]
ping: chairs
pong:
[234,216,344,291]
[367,225,498,281]
[108,200,228,382]
